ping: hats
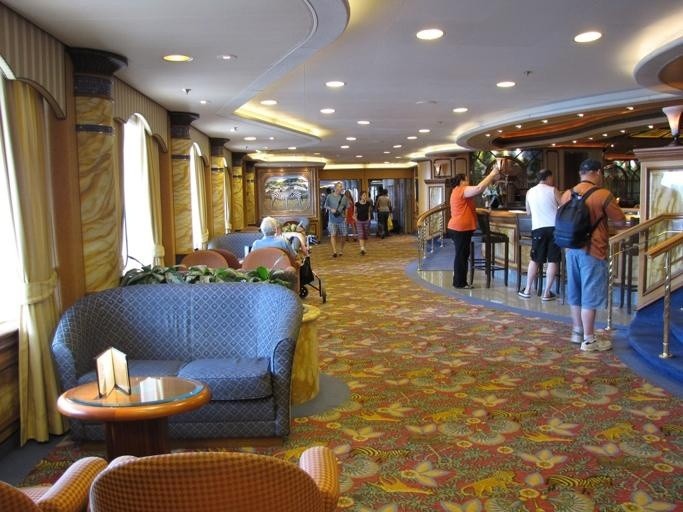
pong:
[577,157,603,173]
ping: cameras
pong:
[334,210,341,217]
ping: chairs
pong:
[88,444,341,512]
[466,208,509,288]
[619,216,650,314]
[243,249,296,277]
[269,246,301,294]
[514,215,560,295]
[204,250,241,270]
[0,457,109,512]
[180,250,229,274]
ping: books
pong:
[92,346,131,398]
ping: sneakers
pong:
[339,251,344,256]
[579,335,613,353]
[332,252,338,258]
[569,328,583,344]
[361,249,365,256]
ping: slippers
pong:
[460,284,474,290]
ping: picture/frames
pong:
[646,165,683,292]
[255,169,317,219]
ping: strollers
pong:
[299,234,327,303]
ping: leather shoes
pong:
[518,287,532,299]
[541,290,557,302]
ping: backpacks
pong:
[555,186,607,249]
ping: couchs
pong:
[51,282,303,441]
[206,233,270,258]
[274,215,309,239]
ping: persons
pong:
[376,189,393,239]
[448,168,500,289]
[250,216,302,270]
[353,191,371,257]
[345,190,357,242]
[324,182,347,257]
[519,169,562,301]
[275,221,308,254]
[374,186,384,205]
[561,158,626,352]
